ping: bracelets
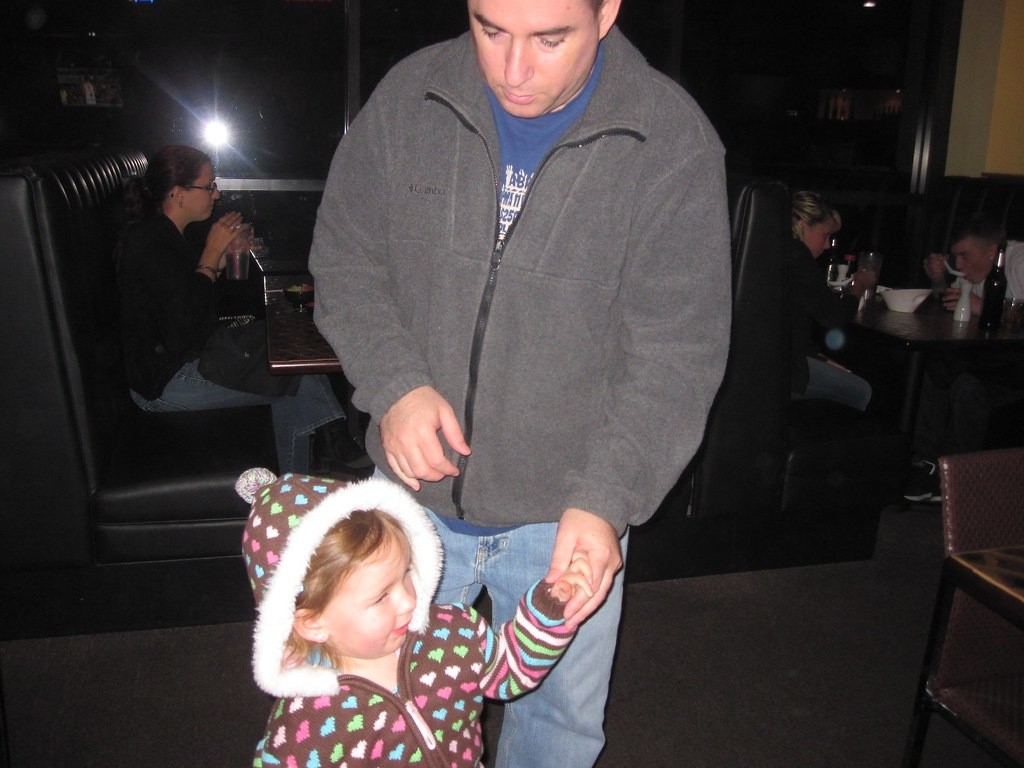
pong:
[195,265,218,282]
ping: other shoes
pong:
[904,483,934,502]
[311,422,374,476]
[931,491,942,502]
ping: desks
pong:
[265,271,348,377]
[847,296,1023,505]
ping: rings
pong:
[231,226,236,229]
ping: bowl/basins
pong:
[841,295,868,310]
[882,290,934,312]
[284,285,315,307]
[939,291,954,314]
[306,301,315,317]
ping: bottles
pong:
[980,240,1009,333]
[955,283,973,323]
[835,264,850,290]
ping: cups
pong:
[226,218,252,281]
[1005,298,1023,326]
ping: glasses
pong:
[186,182,215,194]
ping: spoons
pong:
[830,274,859,287]
[942,256,968,276]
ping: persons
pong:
[235,467,594,768]
[308,0,732,768]
[788,190,873,411]
[903,211,1024,501]
[111,145,347,475]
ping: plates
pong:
[876,285,890,293]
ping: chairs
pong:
[902,447,1024,768]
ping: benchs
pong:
[624,175,1024,586]
[0,149,275,642]
